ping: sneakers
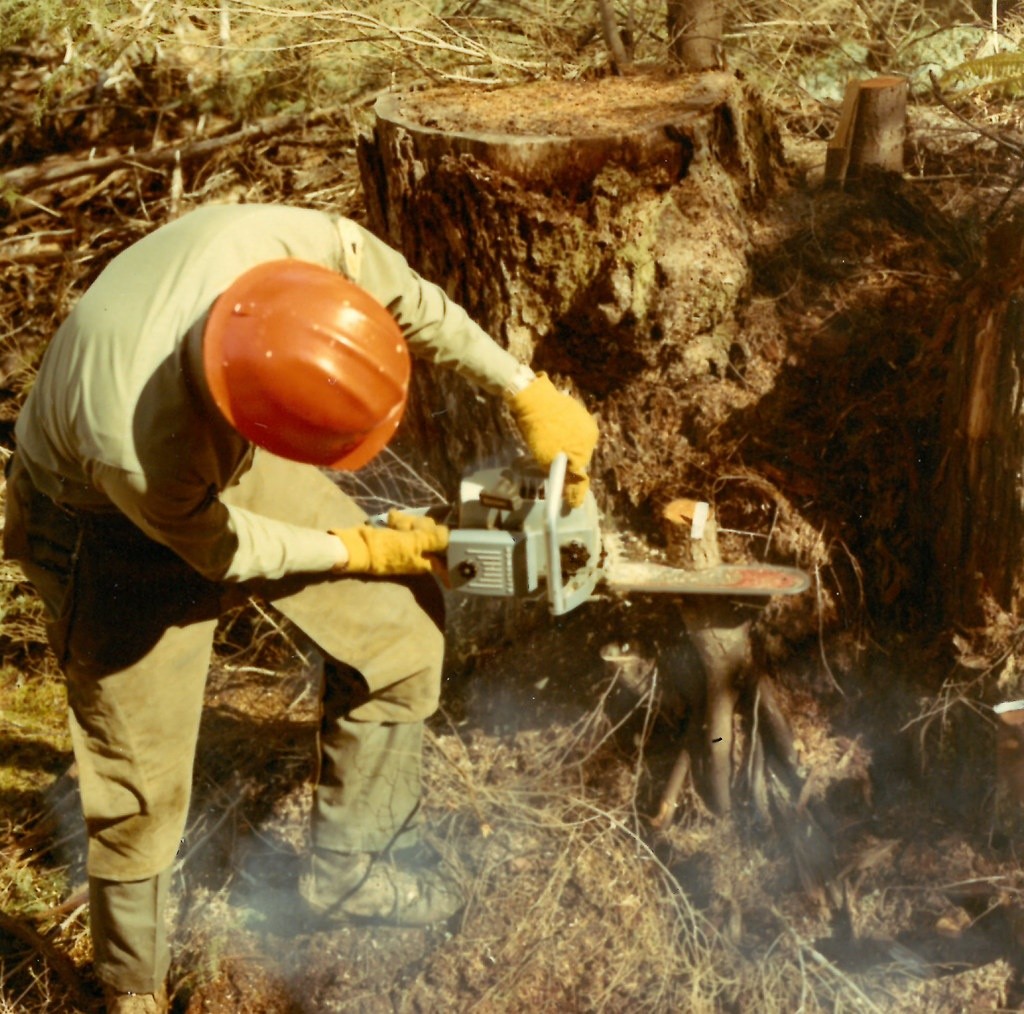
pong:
[300,849,469,925]
[103,986,164,1014]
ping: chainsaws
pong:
[368,449,813,614]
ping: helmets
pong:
[201,259,409,473]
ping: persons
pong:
[4,204,599,1014]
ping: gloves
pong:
[328,506,448,576]
[510,370,603,509]
